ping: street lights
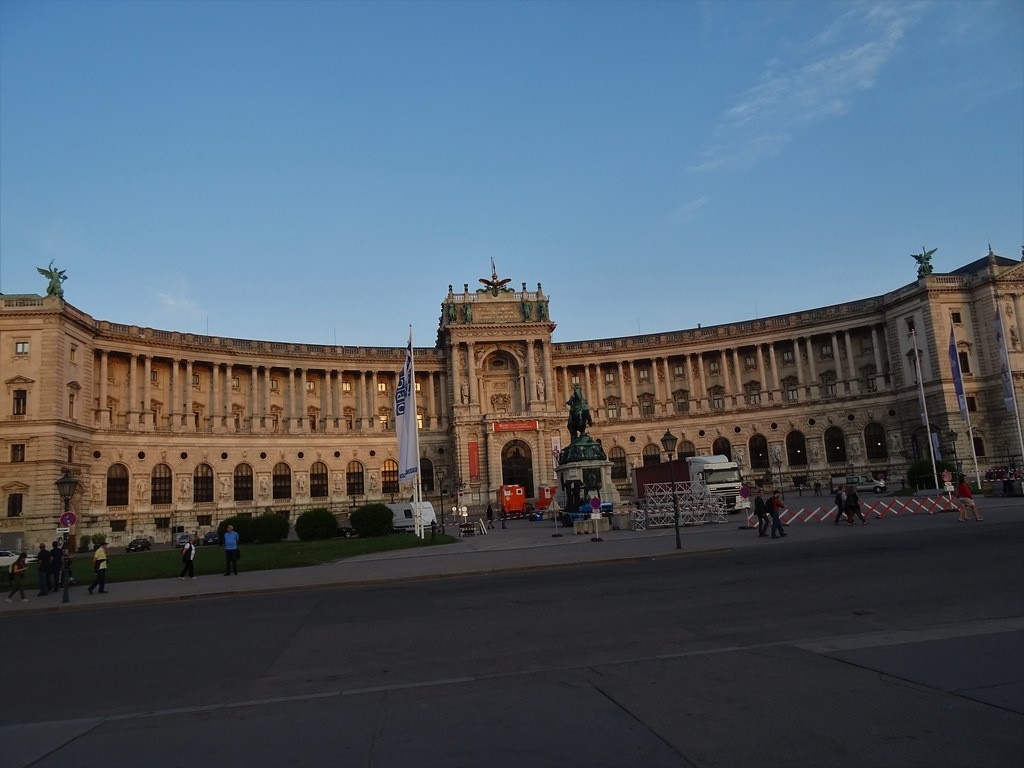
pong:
[947,428,961,484]
[53,468,79,604]
[169,512,175,546]
[423,484,428,502]
[659,428,682,549]
[775,459,785,501]
[436,470,447,535]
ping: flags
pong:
[948,325,969,421]
[395,339,419,482]
[915,338,929,426]
[995,306,1016,411]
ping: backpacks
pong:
[835,491,843,505]
[765,497,774,513]
[8,561,20,577]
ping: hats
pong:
[100,541,109,546]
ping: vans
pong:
[384,501,437,531]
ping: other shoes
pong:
[4,597,14,603]
[833,522,840,526]
[234,572,238,575]
[88,587,95,595]
[771,535,780,539]
[190,577,196,579]
[22,599,30,604]
[224,572,231,576]
[98,590,108,594]
[847,522,854,527]
[179,576,186,580]
[779,533,788,537]
[863,520,869,525]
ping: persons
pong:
[464,299,475,321]
[448,301,457,322]
[88,542,110,595]
[901,474,906,489]
[754,490,787,539]
[223,525,239,577]
[812,480,822,496]
[799,484,805,496]
[34,540,62,597]
[185,529,199,535]
[957,473,983,521]
[834,486,855,525]
[6,553,31,603]
[538,295,550,319]
[566,388,593,426]
[179,536,197,580]
[520,296,532,319]
[48,261,64,292]
[485,504,508,530]
[846,485,868,526]
[553,446,560,467]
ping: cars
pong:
[175,534,201,548]
[0,550,39,566]
[125,539,152,553]
[203,532,221,546]
[334,526,356,538]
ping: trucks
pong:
[495,484,526,518]
[831,475,888,494]
[630,455,750,516]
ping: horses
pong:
[565,385,593,443]
